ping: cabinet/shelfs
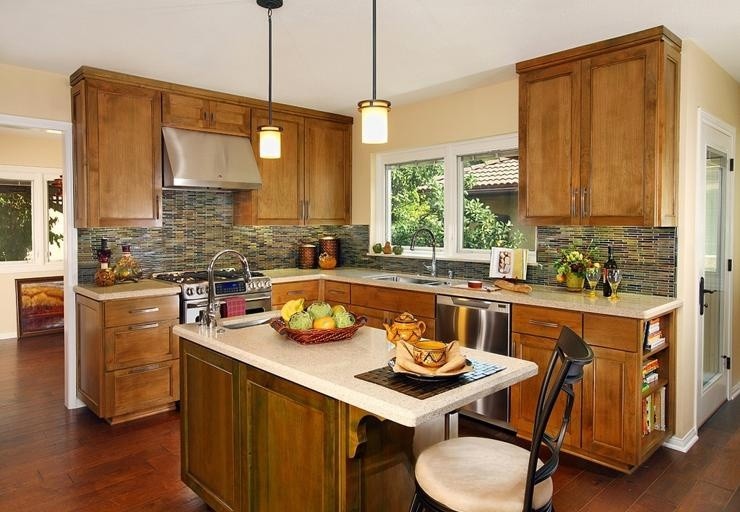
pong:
[510,304,678,477]
[235,98,353,226]
[322,276,437,340]
[272,277,322,310]
[162,82,251,136]
[70,65,162,229]
[73,293,181,425]
[515,25,680,228]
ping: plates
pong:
[388,357,471,383]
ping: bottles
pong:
[603,246,618,296]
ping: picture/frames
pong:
[15,276,64,339]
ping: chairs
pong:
[411,324,595,512]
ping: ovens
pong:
[178,292,272,323]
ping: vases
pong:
[566,272,586,291]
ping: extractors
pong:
[161,126,262,194]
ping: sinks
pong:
[373,275,445,286]
[430,281,464,285]
[223,316,274,329]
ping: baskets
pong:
[269,311,368,346]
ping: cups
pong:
[411,341,449,367]
[468,280,482,289]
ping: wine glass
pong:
[586,268,601,296]
[607,268,622,299]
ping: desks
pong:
[173,310,539,511]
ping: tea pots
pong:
[382,312,426,344]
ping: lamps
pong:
[257,0,282,160]
[357,0,390,144]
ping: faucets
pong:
[207,249,252,327]
[410,229,436,276]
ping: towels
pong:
[225,296,246,317]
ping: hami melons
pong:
[288,311,313,333]
[336,314,355,328]
[308,302,333,319]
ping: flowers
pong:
[553,240,601,282]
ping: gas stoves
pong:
[150,268,271,293]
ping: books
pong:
[642,387,667,436]
[642,357,659,392]
[643,317,665,350]
[488,247,528,281]
